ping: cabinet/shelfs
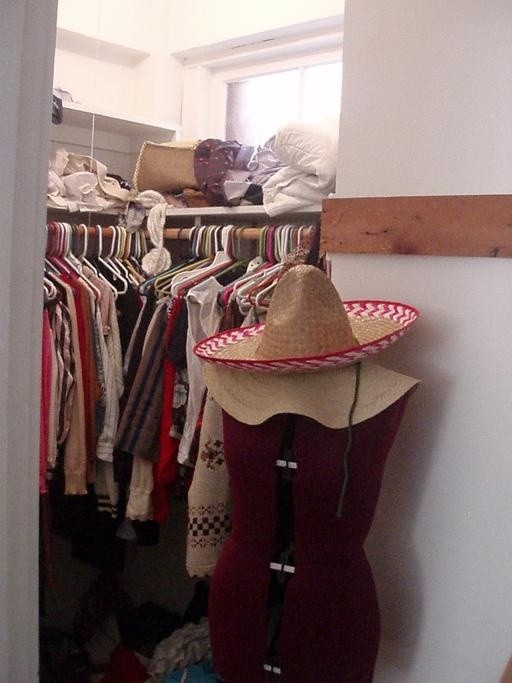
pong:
[47,97,174,216]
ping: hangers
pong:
[139,224,320,315]
[44,221,147,302]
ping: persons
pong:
[203,346,420,682]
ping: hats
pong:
[194,264,419,373]
